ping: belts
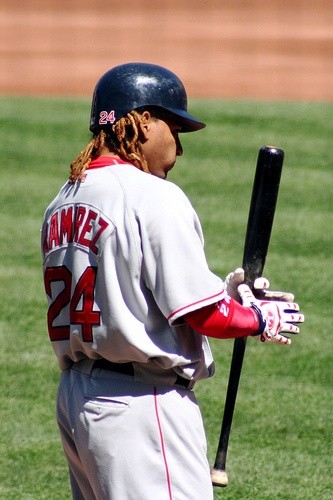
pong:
[92,357,190,387]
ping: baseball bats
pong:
[210,146,283,486]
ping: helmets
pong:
[89,62,205,137]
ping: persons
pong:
[42,63,305,499]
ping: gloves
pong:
[235,283,304,344]
[223,269,295,306]
